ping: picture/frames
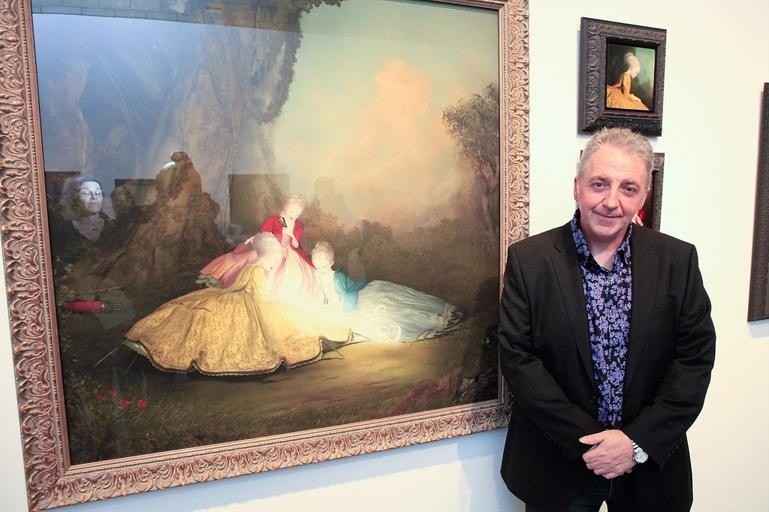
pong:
[0,1,530,512]
[577,17,666,137]
[747,82,768,322]
[580,149,664,232]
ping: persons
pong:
[59,177,150,276]
[496,126,716,512]
[604,51,651,112]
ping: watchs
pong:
[630,439,649,464]
